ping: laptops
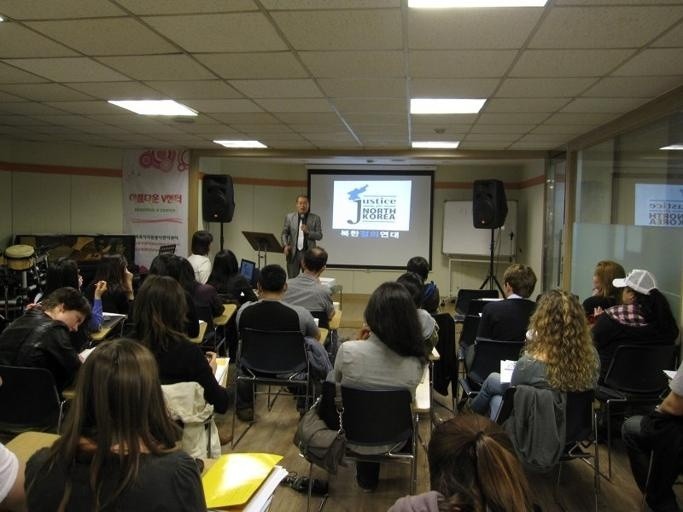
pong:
[238,258,255,285]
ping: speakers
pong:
[472,179,508,229]
[454,289,499,316]
[201,174,234,223]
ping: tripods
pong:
[479,229,506,299]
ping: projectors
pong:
[318,277,336,288]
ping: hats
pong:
[613,269,659,294]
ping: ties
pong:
[296,214,304,251]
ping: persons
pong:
[388,415,540,512]
[130,275,231,414]
[24,338,207,512]
[38,246,335,379]
[186,231,214,284]
[581,260,680,337]
[621,362,683,512]
[332,282,431,496]
[469,288,601,423]
[279,194,322,279]
[397,255,440,351]
[0,443,30,512]
[476,263,538,342]
[0,287,91,434]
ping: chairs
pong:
[457,336,533,414]
[458,298,496,348]
[492,385,603,512]
[632,413,682,512]
[4,256,237,512]
[453,286,500,326]
[234,299,318,435]
[426,312,459,414]
[304,361,432,510]
[593,343,680,478]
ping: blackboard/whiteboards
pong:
[441,199,518,257]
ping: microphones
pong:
[300,214,305,225]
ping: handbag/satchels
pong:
[291,383,348,475]
